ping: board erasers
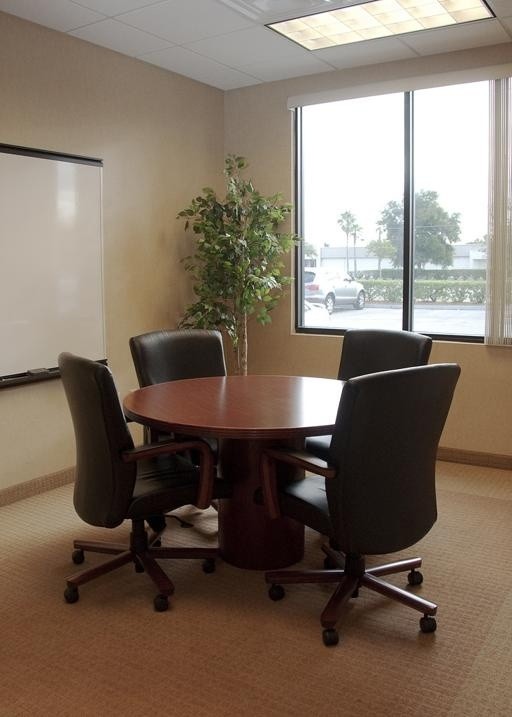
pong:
[27,368,50,376]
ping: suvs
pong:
[305,266,366,314]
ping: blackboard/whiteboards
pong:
[0,143,109,389]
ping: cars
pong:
[304,300,329,327]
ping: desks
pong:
[119,374,351,574]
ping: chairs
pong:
[129,330,238,511]
[302,327,439,467]
[258,358,459,647]
[57,346,216,616]
